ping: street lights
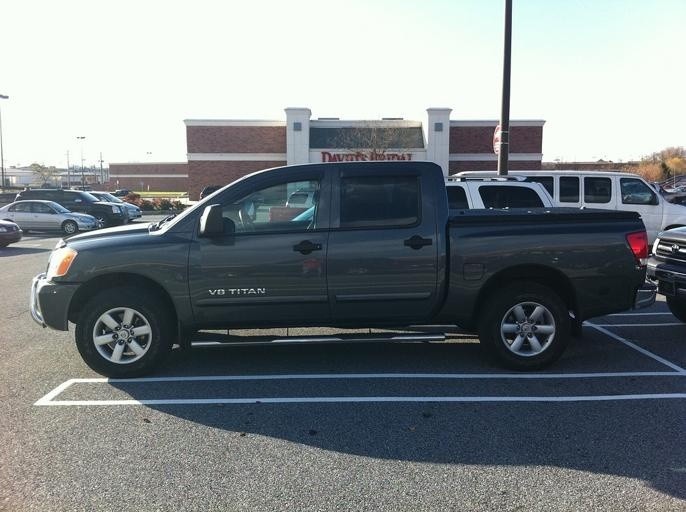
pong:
[75,135,86,186]
[0,92,11,190]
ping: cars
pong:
[0,187,142,250]
